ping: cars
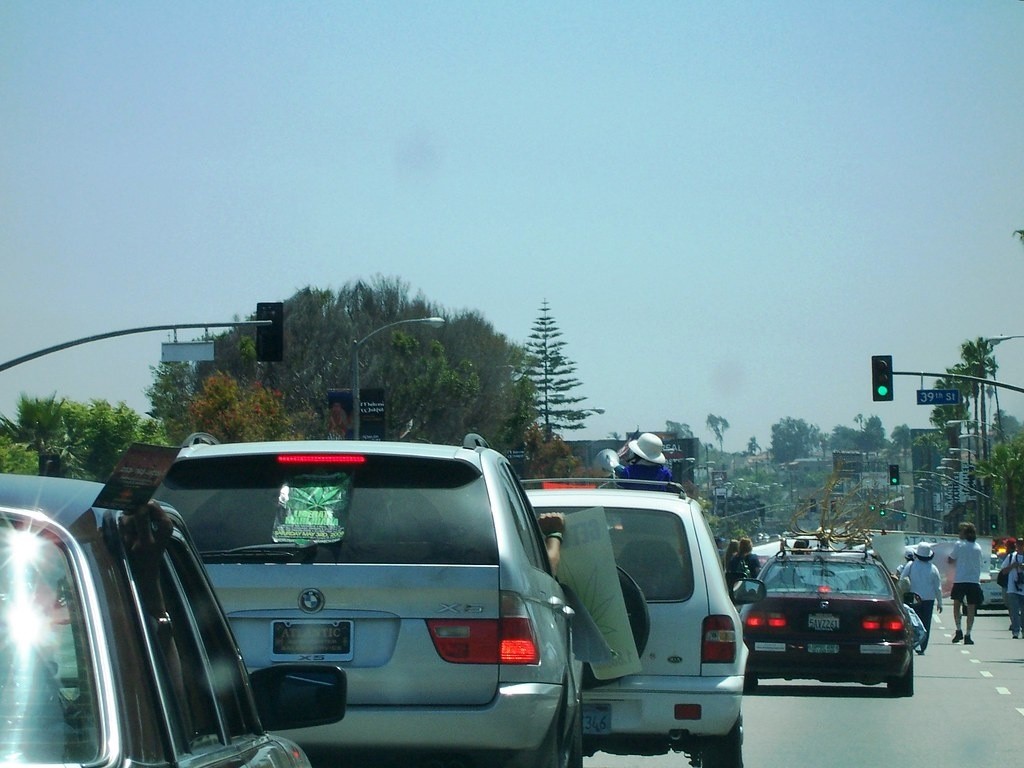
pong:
[0,430,768,768]
[739,547,923,695]
[960,554,1010,615]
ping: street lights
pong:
[350,316,447,441]
[902,419,1009,536]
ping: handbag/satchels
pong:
[996,569,1008,587]
[899,576,911,592]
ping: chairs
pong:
[846,576,873,591]
[766,568,802,587]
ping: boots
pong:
[963,634,973,645]
[952,629,963,643]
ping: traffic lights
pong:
[879,502,886,517]
[871,355,894,403]
[991,514,998,530]
[889,464,900,485]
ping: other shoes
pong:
[1009,624,1012,629]
[1013,635,1018,639]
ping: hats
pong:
[628,433,665,464]
[1016,537,1024,544]
[794,539,809,554]
[1002,537,1016,545]
[913,542,933,557]
[904,550,914,559]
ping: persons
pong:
[897,541,944,655]
[614,433,672,492]
[715,536,763,597]
[538,512,565,577]
[997,537,1024,640]
[947,523,984,645]
[775,534,808,556]
